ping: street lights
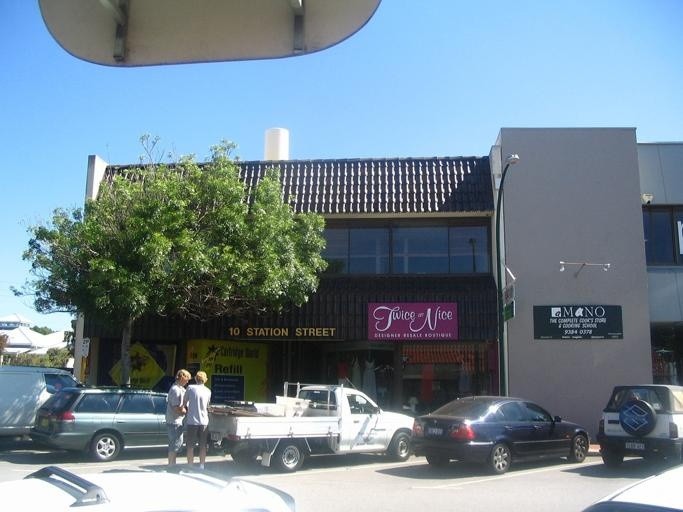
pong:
[494,152,521,396]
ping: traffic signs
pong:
[502,283,515,302]
[503,300,514,321]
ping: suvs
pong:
[29,386,230,463]
[594,384,683,468]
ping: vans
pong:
[0,366,86,437]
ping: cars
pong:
[409,396,590,475]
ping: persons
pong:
[182,370,214,470]
[162,367,191,470]
[335,352,395,408]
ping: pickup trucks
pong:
[207,383,417,472]
